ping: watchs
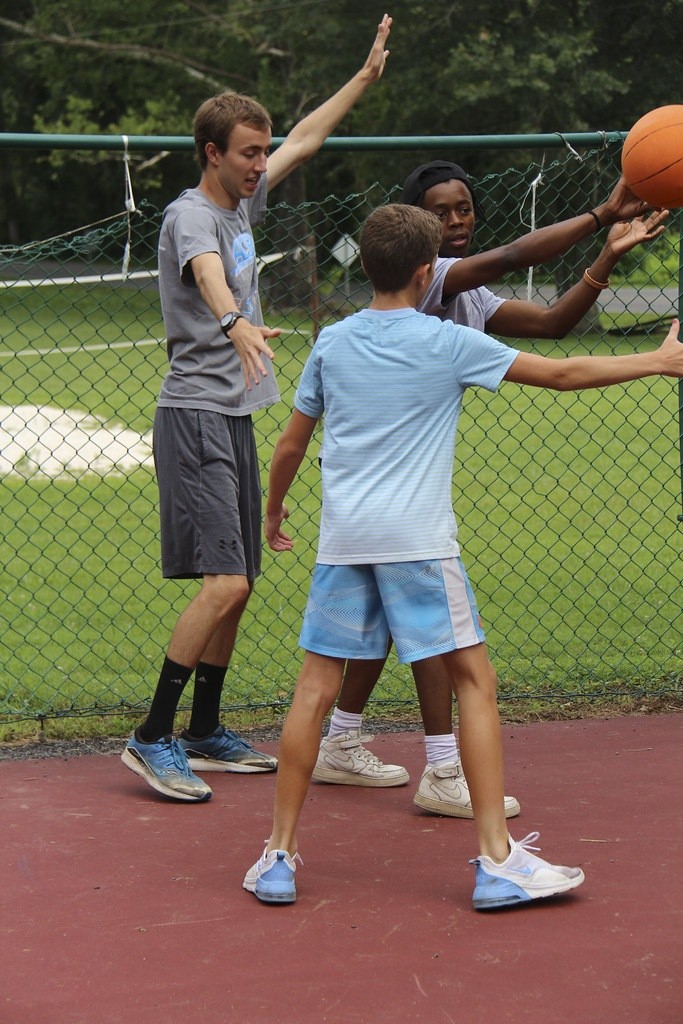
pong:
[220,311,245,340]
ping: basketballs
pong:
[622,104,682,208]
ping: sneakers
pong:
[176,725,279,773]
[121,723,212,801]
[467,832,585,908]
[412,761,521,819]
[241,835,304,903]
[311,730,410,787]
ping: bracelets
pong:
[584,268,611,290]
[587,210,601,232]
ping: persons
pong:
[238,205,682,913]
[119,14,393,803]
[312,159,669,822]
